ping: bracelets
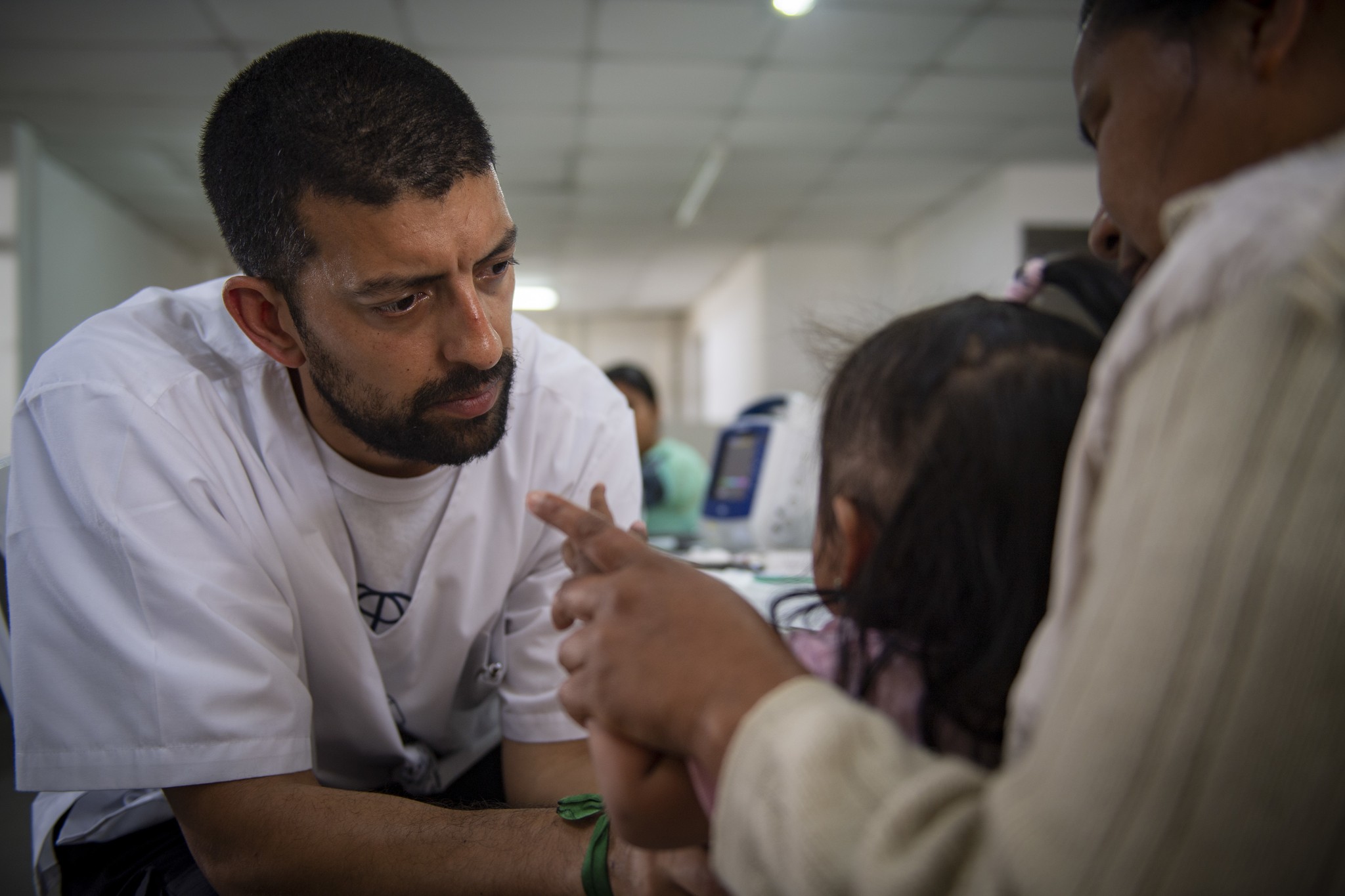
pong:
[556,794,614,896]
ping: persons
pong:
[562,248,1141,853]
[602,366,714,539]
[525,2,1345,896]
[2,29,739,896]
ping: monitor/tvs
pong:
[696,390,807,547]
